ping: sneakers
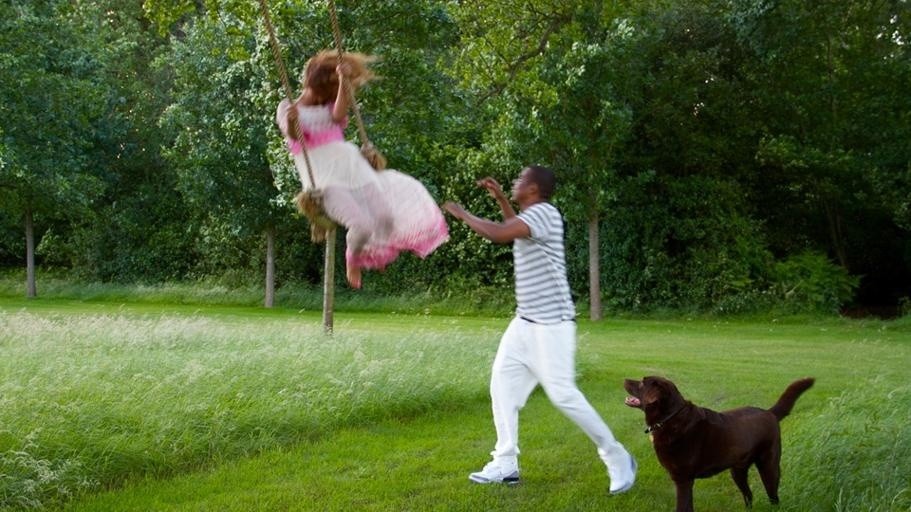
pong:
[468,457,519,484]
[598,442,638,494]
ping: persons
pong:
[441,165,639,495]
[276,48,452,290]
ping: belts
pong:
[520,316,575,324]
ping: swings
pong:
[260,0,385,230]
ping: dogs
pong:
[620,373,816,512]
